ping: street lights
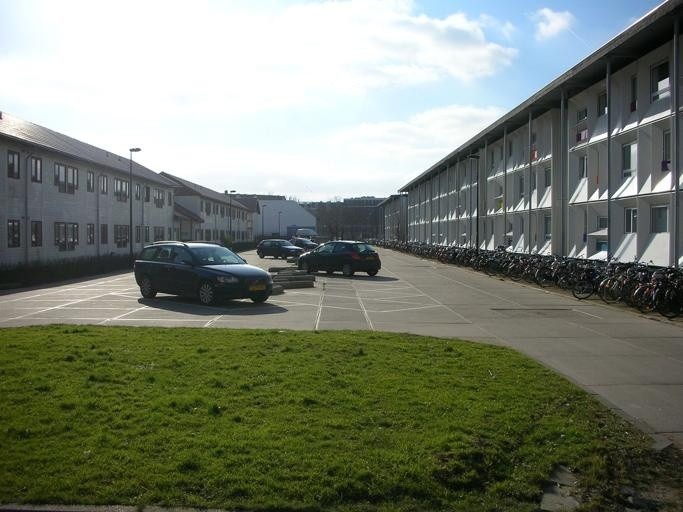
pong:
[129,148,141,270]
[230,191,236,247]
[278,211,283,237]
[262,204,267,239]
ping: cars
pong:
[290,237,318,251]
[296,240,381,277]
[257,238,303,260]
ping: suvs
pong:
[133,241,274,306]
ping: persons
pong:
[289,236,296,245]
[310,235,315,242]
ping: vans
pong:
[296,229,319,235]
